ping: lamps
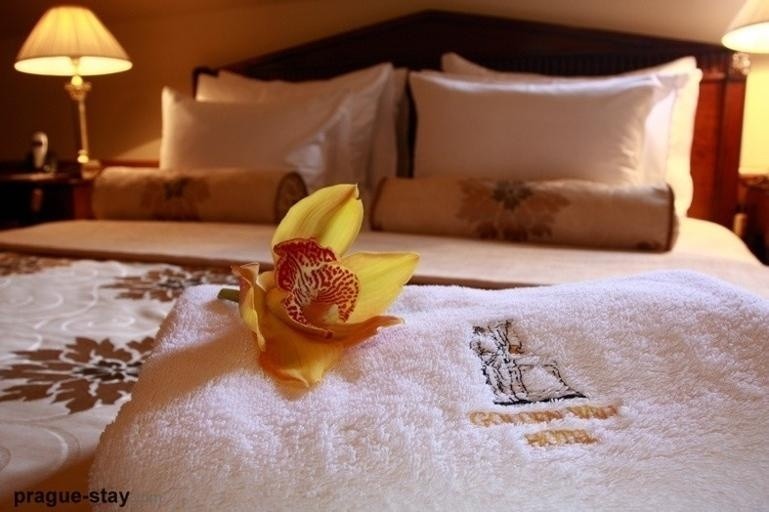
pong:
[13,6,133,181]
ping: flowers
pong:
[216,182,420,389]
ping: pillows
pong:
[94,53,702,252]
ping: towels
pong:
[85,267,766,510]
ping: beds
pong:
[0,8,768,512]
